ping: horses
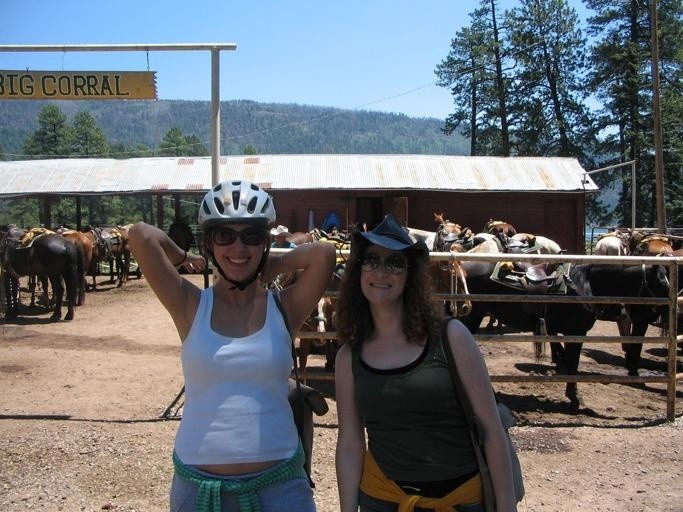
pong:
[285,211,683,413]
[1,223,142,323]
[168,214,193,252]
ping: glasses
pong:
[212,228,264,245]
[359,252,407,273]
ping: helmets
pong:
[197,179,277,226]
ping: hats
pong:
[357,214,429,251]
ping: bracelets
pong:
[172,251,187,268]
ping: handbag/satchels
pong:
[468,408,525,512]
[289,378,329,488]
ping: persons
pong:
[128,178,338,511]
[333,214,524,510]
[268,225,298,290]
